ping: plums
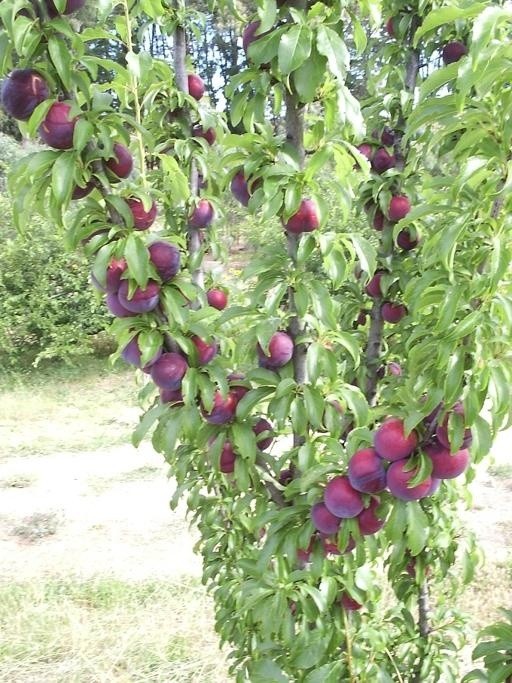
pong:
[1,0,475,611]
